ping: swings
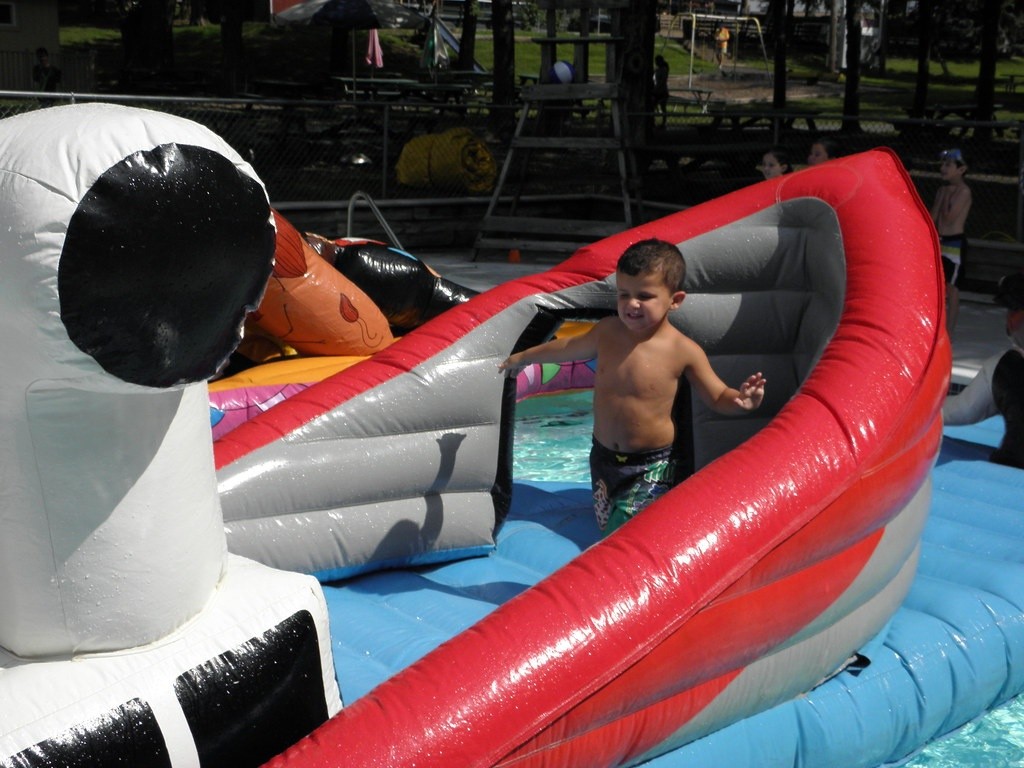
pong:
[700,19,720,82]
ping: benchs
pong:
[687,124,771,127]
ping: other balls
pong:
[550,60,575,84]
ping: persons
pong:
[499,238,766,536]
[31,48,61,107]
[655,23,1024,468]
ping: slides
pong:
[432,17,487,73]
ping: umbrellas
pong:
[365,29,384,77]
[421,21,448,83]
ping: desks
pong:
[1000,74,1024,95]
[899,104,1004,137]
[331,71,822,138]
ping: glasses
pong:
[929,149,966,164]
